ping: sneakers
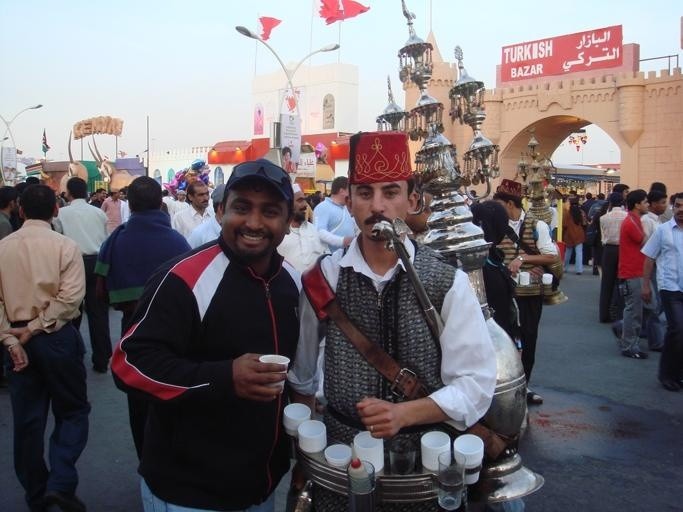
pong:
[39,488,87,511]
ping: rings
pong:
[371,425,375,432]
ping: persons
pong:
[492,180,560,406]
[406,175,433,244]
[1,184,93,512]
[110,158,308,512]
[545,181,683,391]
[284,131,496,511]
[276,174,359,276]
[2,176,229,374]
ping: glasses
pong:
[228,163,291,183]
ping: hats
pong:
[290,183,301,195]
[222,159,293,202]
[349,131,412,185]
[210,183,226,204]
[495,178,526,199]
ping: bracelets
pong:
[517,255,524,263]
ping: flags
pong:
[318,0,370,26]
[259,16,281,40]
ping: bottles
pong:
[348,458,370,483]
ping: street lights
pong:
[235,24,340,183]
[0,103,43,186]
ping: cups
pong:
[259,354,291,398]
[511,268,553,286]
[388,438,418,476]
[296,421,326,454]
[282,403,311,439]
[346,461,375,493]
[437,451,467,512]
[454,434,484,487]
[420,430,452,472]
[351,431,387,474]
[322,442,356,467]
